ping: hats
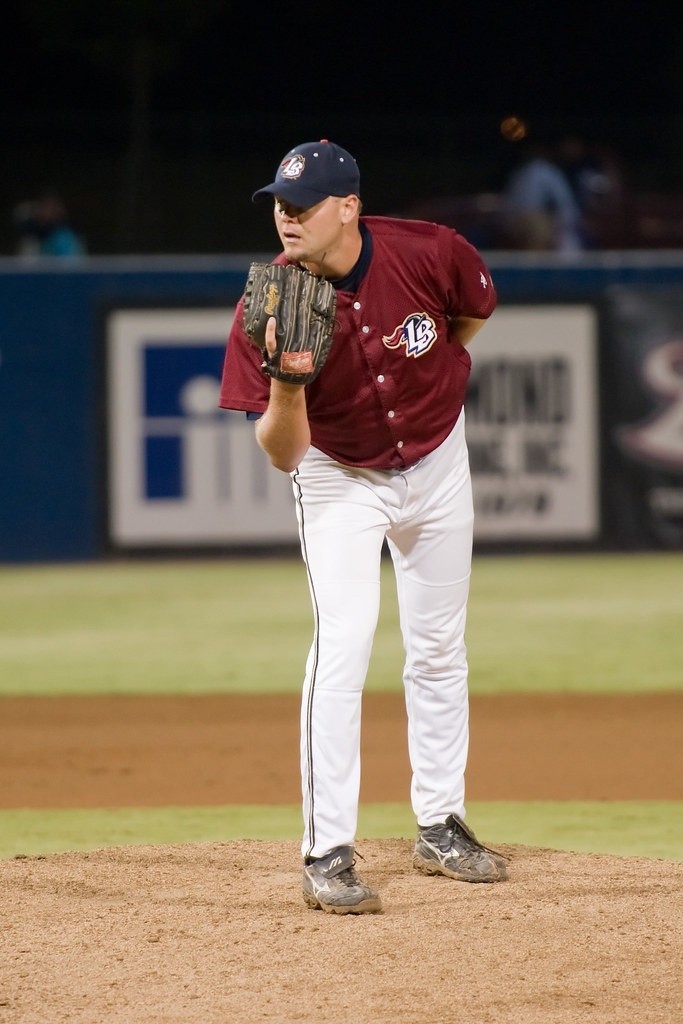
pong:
[252,139,361,209]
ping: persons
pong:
[15,187,87,257]
[505,158,586,251]
[219,140,511,915]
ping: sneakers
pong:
[413,813,512,883]
[301,846,382,914]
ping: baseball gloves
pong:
[241,251,338,383]
